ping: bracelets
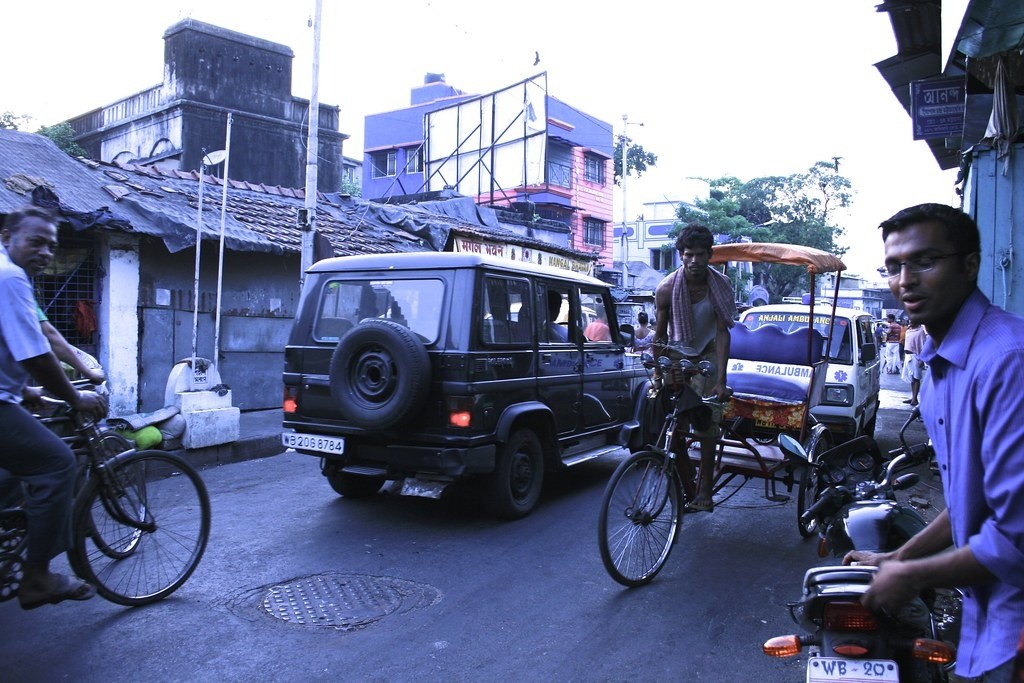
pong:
[654,376,661,381]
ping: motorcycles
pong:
[762,403,967,683]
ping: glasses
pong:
[877,251,968,279]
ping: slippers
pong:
[688,495,713,511]
[19,573,97,610]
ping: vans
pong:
[736,302,882,444]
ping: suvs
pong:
[279,250,658,521]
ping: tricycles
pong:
[594,350,831,590]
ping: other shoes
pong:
[903,399,912,403]
[911,400,918,405]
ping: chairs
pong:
[723,321,826,433]
[318,317,604,344]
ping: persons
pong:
[390,301,411,319]
[489,287,518,341]
[653,223,733,509]
[902,319,926,406]
[883,314,900,374]
[546,290,567,342]
[633,311,655,352]
[899,318,906,367]
[647,317,656,330]
[841,202,1024,682]
[875,323,888,353]
[0,206,111,612]
[36,294,102,382]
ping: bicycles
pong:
[28,376,147,559]
[1,399,213,607]
